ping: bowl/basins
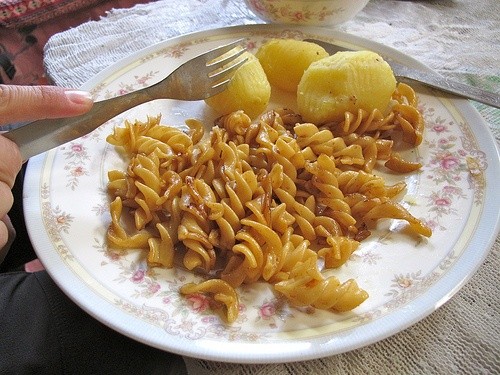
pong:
[244,0,369,28]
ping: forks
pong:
[2,38,248,162]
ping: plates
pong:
[22,24,500,365]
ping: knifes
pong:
[303,39,500,108]
